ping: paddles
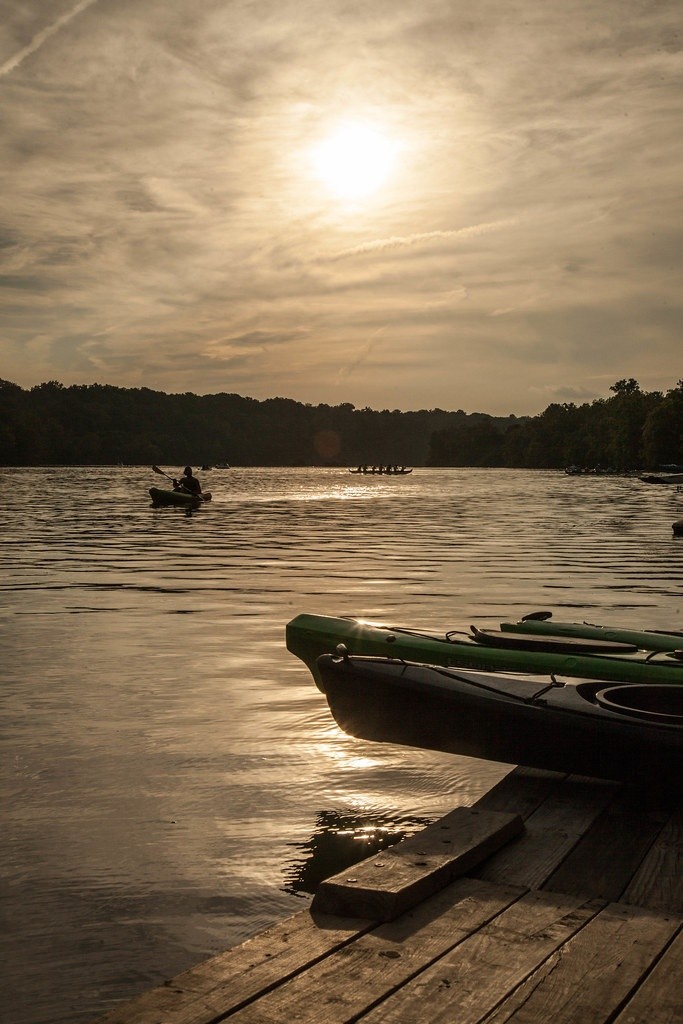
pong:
[152,465,206,502]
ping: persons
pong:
[394,464,398,471]
[594,464,600,472]
[358,465,362,471]
[363,464,367,471]
[585,465,590,472]
[402,464,406,471]
[173,467,201,494]
[386,464,391,470]
[372,465,376,471]
[570,464,575,473]
[576,464,582,473]
[379,464,384,471]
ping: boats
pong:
[216,465,229,469]
[565,468,644,476]
[349,469,414,474]
[148,487,212,506]
[285,612,683,796]
[638,475,683,484]
[199,468,212,471]
